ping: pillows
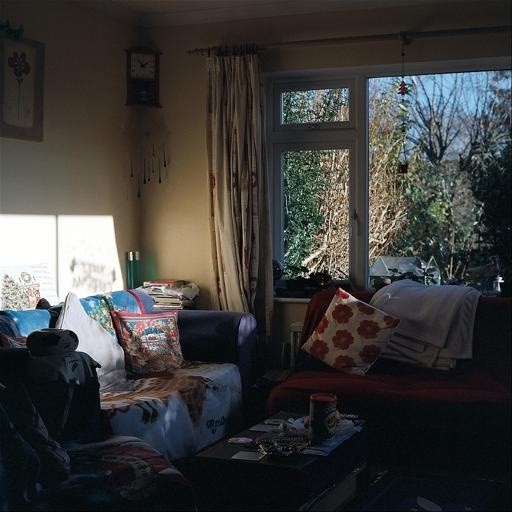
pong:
[299,287,401,378]
[111,309,188,379]
[53,291,127,393]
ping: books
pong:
[140,278,188,310]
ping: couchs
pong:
[0,289,261,512]
[264,286,512,417]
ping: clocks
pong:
[123,43,164,109]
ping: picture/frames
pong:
[0,34,46,142]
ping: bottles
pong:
[125,250,143,289]
